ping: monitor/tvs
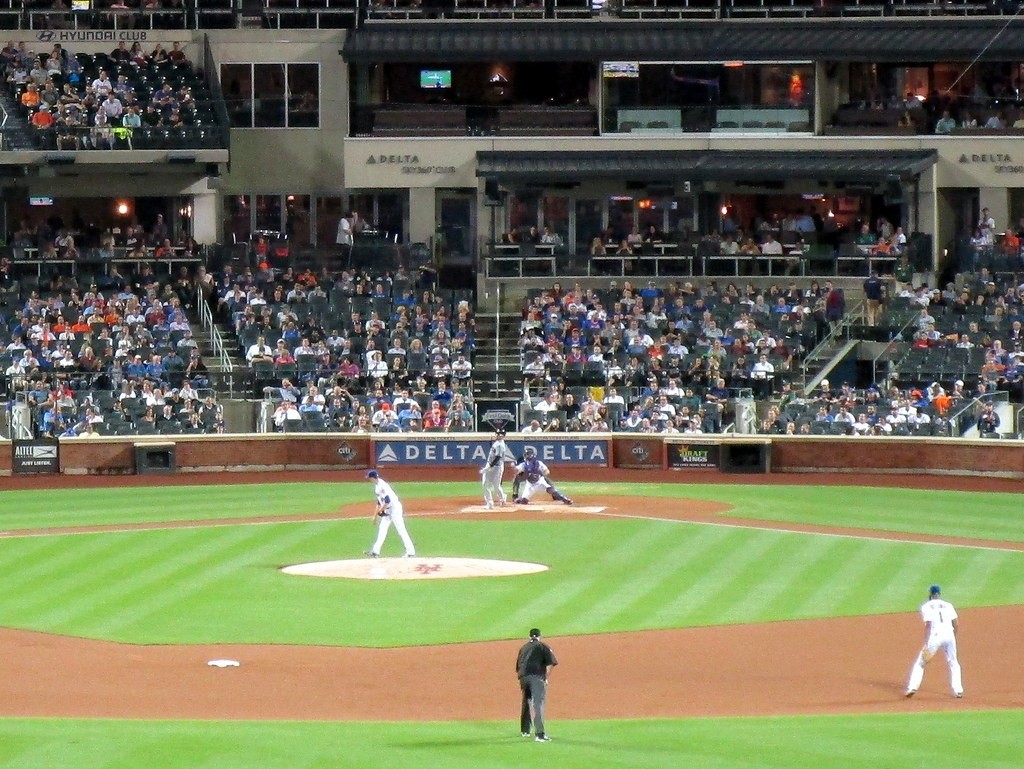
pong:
[29,197,54,207]
[420,70,451,89]
[72,0,90,10]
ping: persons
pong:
[858,78,1024,134]
[0,39,191,150]
[905,584,963,698]
[479,427,507,509]
[516,629,558,742]
[510,448,574,505]
[365,470,414,557]
[0,208,1024,441]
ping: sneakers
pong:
[522,732,529,736]
[536,735,552,741]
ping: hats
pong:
[929,585,939,593]
[530,629,541,637]
[366,471,378,478]
[27,255,1012,432]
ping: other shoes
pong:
[955,694,961,697]
[563,497,572,504]
[364,551,379,557]
[403,554,413,558]
[906,689,914,697]
[499,501,504,506]
[485,505,493,510]
[516,498,528,503]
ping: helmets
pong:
[496,428,506,435]
[523,448,536,459]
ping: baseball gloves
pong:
[920,648,933,669]
[378,510,390,517]
[527,472,539,484]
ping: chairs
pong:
[498,230,1024,438]
[0,52,220,149]
[0,239,475,434]
